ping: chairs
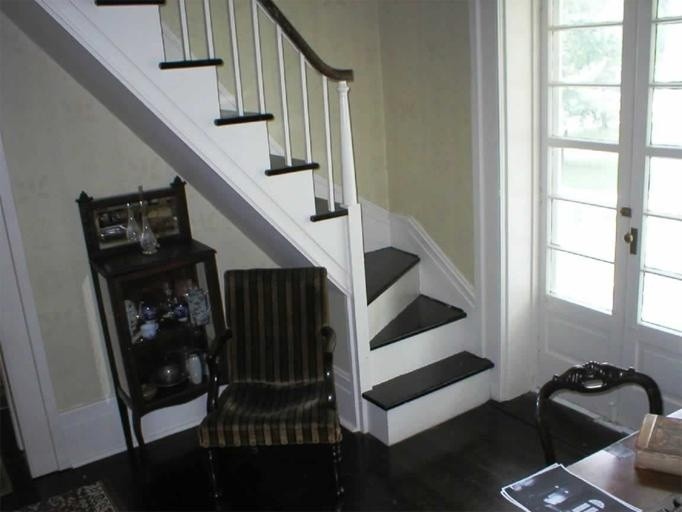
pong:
[199,267,348,509]
[534,359,664,471]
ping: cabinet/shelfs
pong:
[75,175,229,460]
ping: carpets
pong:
[7,478,126,511]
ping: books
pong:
[633,413,682,476]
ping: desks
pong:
[510,406,682,512]
